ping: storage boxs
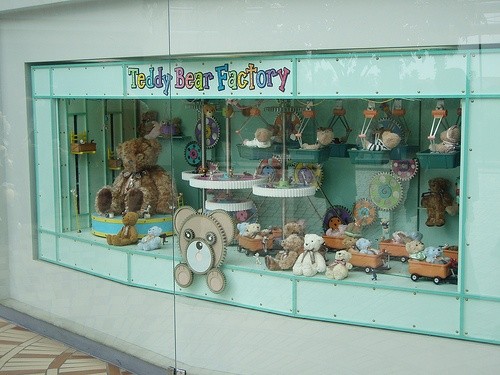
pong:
[236,143,281,160]
[322,235,352,251]
[348,148,390,167]
[70,143,96,152]
[328,143,355,159]
[287,147,327,165]
[237,234,274,251]
[162,126,181,137]
[349,253,384,270]
[442,249,458,261]
[416,148,461,170]
[259,227,282,238]
[381,239,410,258]
[407,258,452,279]
[389,145,418,162]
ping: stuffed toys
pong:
[138,111,182,139]
[392,231,450,264]
[236,222,380,280]
[363,131,401,151]
[429,127,460,153]
[138,226,162,251]
[106,211,138,245]
[243,128,282,149]
[425,178,454,227]
[301,130,334,150]
[95,136,177,216]
[204,104,216,117]
